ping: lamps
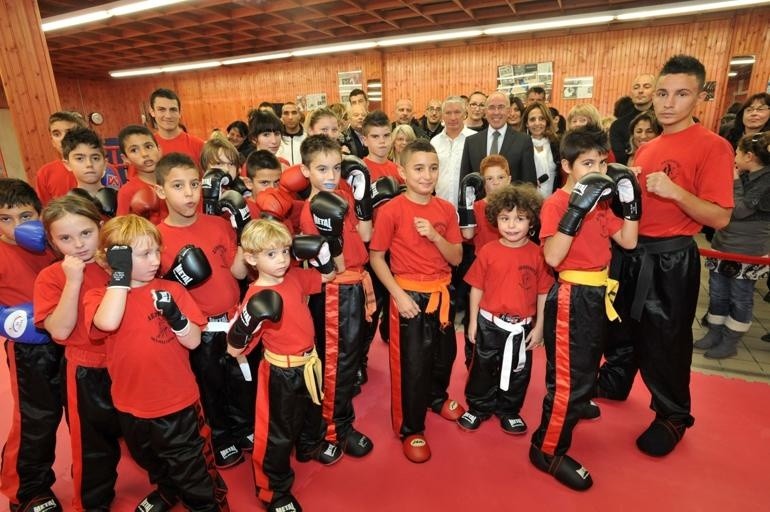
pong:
[106,2,770,79]
[40,1,192,34]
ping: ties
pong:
[489,130,502,154]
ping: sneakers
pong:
[135,488,178,511]
[636,418,686,459]
[402,400,527,462]
[530,442,594,491]
[582,398,601,419]
[213,431,374,512]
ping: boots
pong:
[693,313,726,351]
[703,316,751,360]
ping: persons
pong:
[3,52,770,493]
[0,176,119,509]
[225,215,347,512]
[30,194,196,511]
[79,214,235,512]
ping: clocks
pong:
[91,110,103,125]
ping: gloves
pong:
[201,152,400,274]
[227,290,282,349]
[150,288,191,336]
[96,187,116,216]
[15,221,46,254]
[607,162,641,222]
[105,244,132,290]
[558,171,617,237]
[164,244,211,290]
[1,303,50,345]
[458,173,486,230]
